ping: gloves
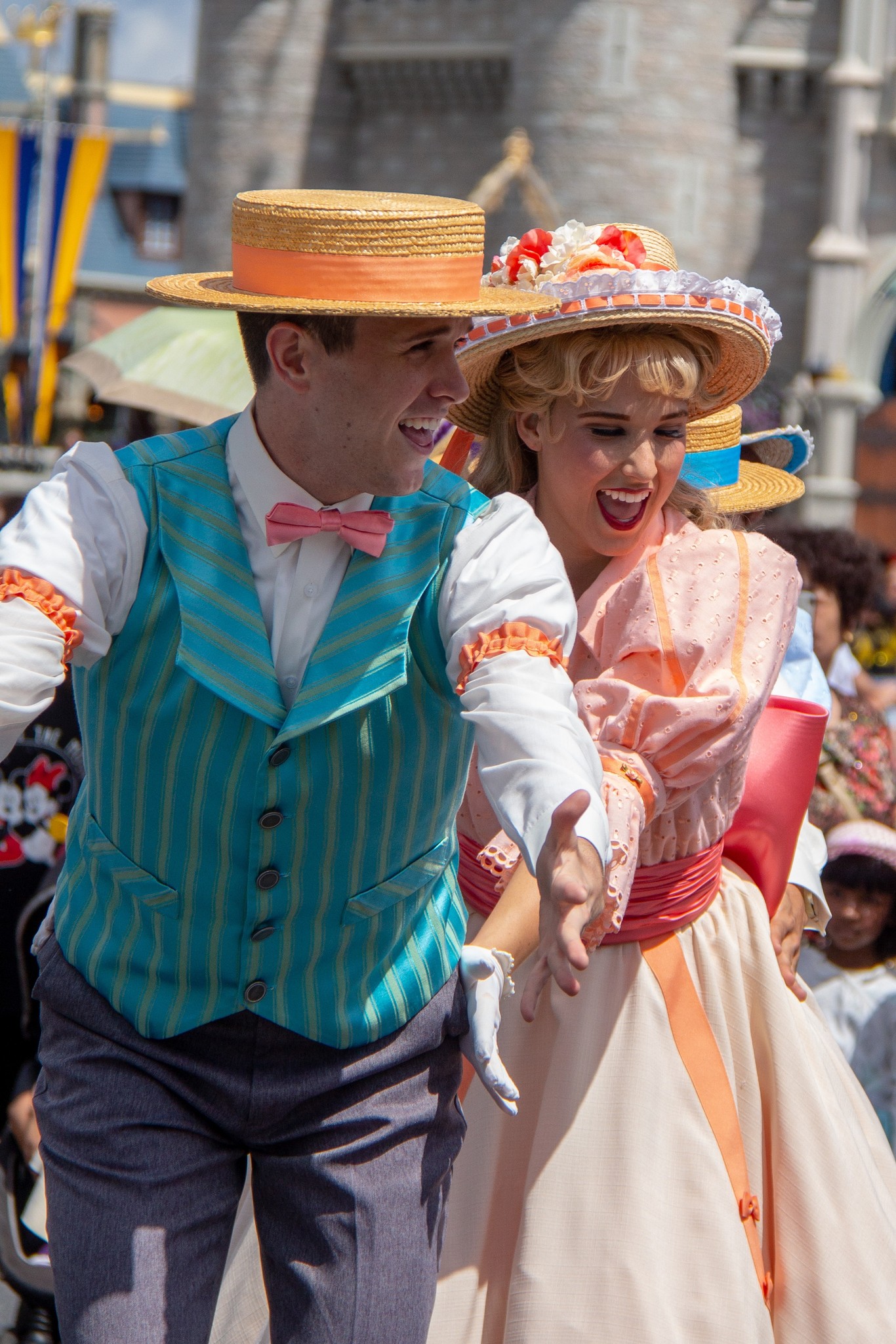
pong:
[458,944,523,1117]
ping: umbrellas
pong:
[60,309,261,430]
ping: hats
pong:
[144,187,561,321]
[677,402,805,516]
[737,423,816,476]
[824,818,896,869]
[443,218,785,437]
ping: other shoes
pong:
[15,1297,62,1344]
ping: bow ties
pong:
[265,501,394,558]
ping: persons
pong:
[457,222,896,1344]
[0,688,88,1344]
[0,187,610,1344]
[684,402,896,1145]
